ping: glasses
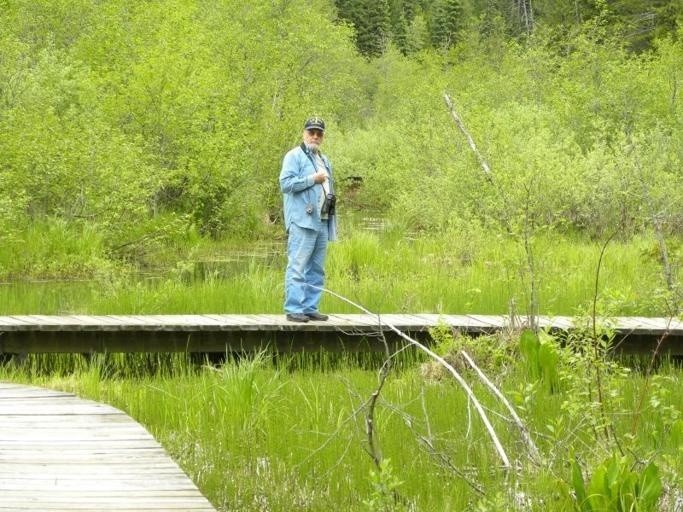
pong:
[309,131,323,137]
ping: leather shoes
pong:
[305,313,328,321]
[287,313,310,322]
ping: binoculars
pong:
[320,194,336,219]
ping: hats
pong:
[304,118,325,131]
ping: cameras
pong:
[321,194,336,215]
[306,204,313,213]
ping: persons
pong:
[279,117,338,322]
